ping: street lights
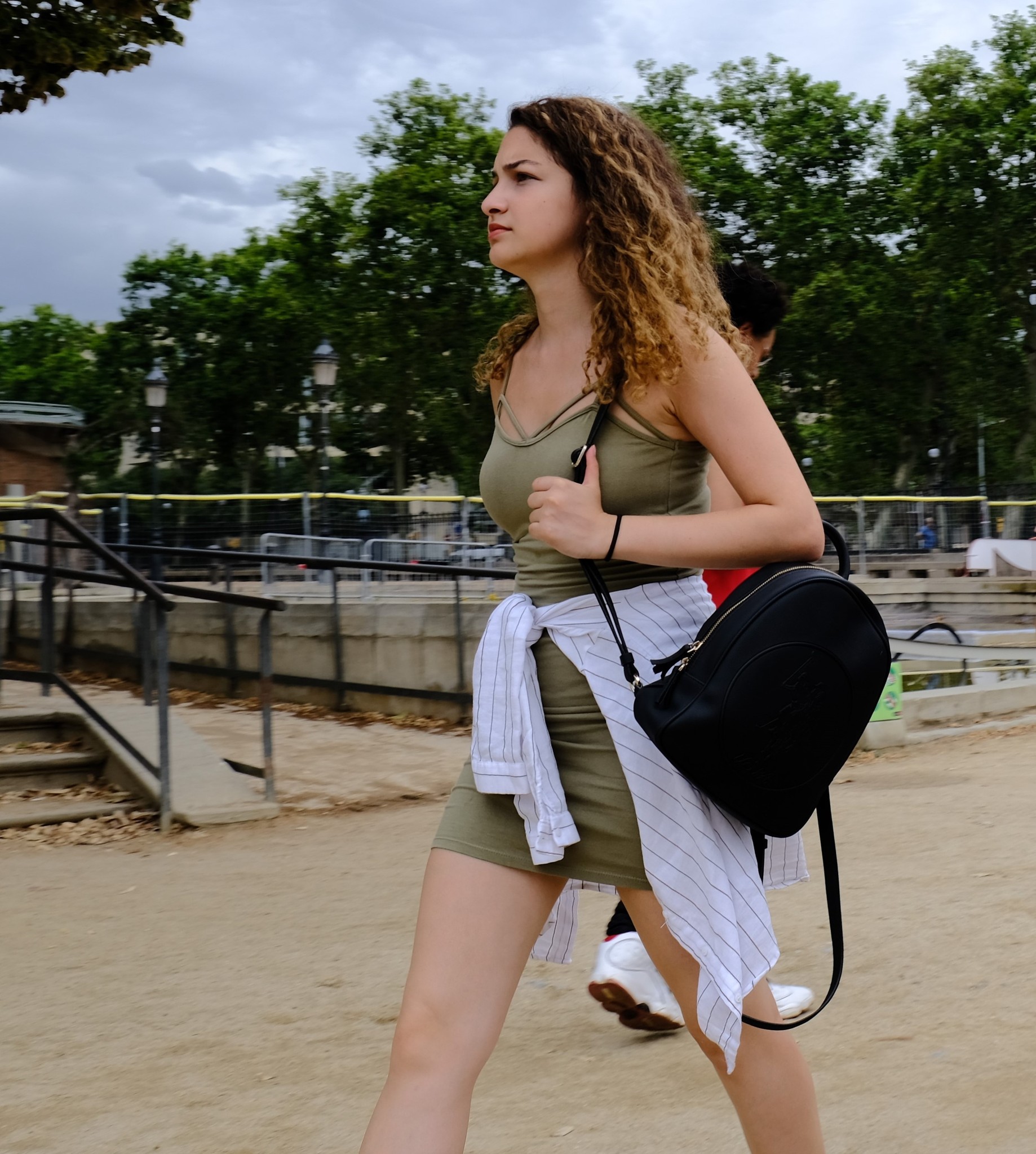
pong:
[927,448,948,553]
[310,345,345,589]
[800,456,813,484]
[140,363,172,586]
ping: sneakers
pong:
[587,930,684,1031]
[767,982,815,1021]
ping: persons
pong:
[359,100,826,1154]
[587,265,816,1031]
[915,516,936,550]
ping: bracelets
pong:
[604,515,623,559]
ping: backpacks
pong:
[571,359,892,1032]
